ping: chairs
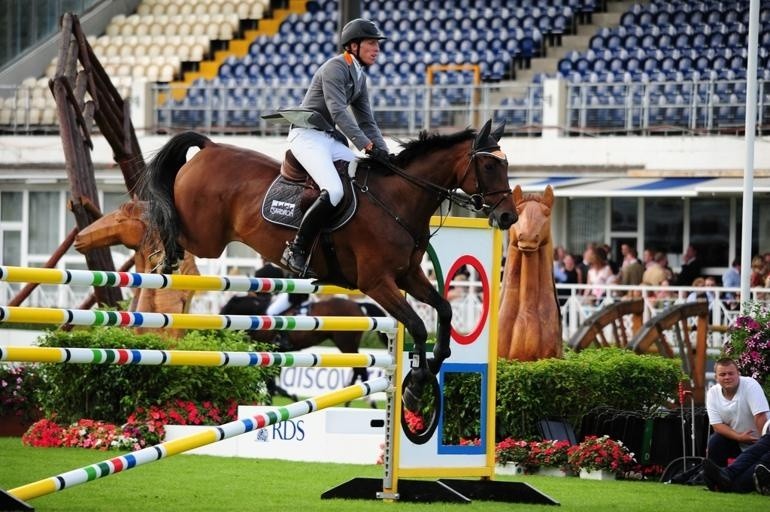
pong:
[480,0,770,135]
[152,1,606,137]
[1,1,283,135]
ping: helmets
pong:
[340,18,388,46]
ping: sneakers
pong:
[699,459,770,496]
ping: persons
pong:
[549,241,770,348]
[264,268,317,350]
[700,433,770,494]
[703,357,770,467]
[254,257,282,298]
[261,16,390,274]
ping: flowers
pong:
[716,298,769,399]
[404,408,426,434]
[375,442,386,465]
[1,359,263,454]
[459,435,639,477]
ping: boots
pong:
[280,189,337,278]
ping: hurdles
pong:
[0,266,406,512]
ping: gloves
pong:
[365,143,391,168]
[383,154,402,177]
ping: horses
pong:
[131,117,518,413]
[67,192,208,344]
[218,294,388,407]
[495,182,565,365]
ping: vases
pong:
[579,467,617,481]
